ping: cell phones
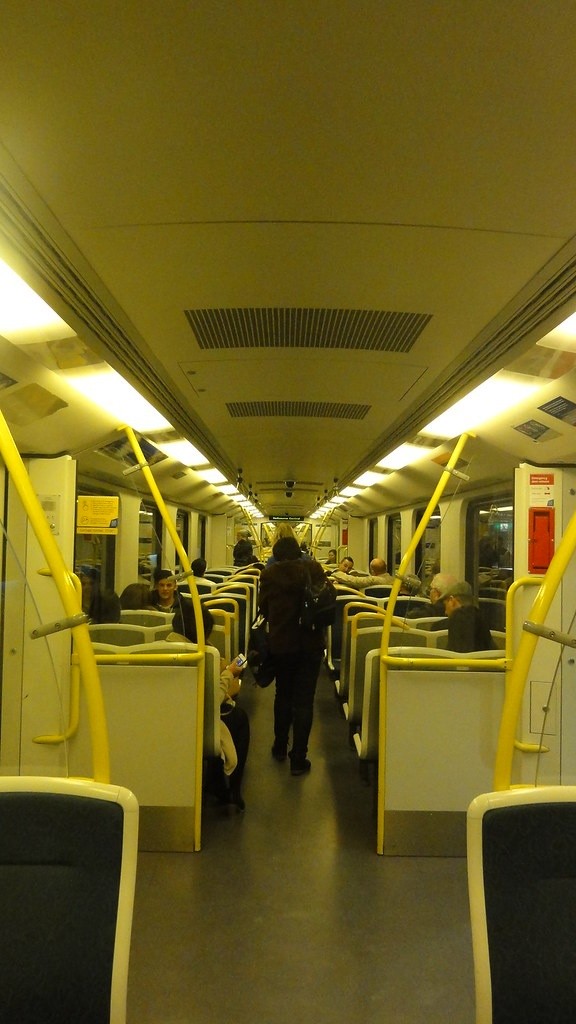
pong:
[225,653,248,670]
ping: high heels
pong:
[226,789,245,818]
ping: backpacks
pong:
[298,558,338,632]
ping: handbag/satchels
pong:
[247,610,277,688]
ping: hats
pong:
[438,581,474,603]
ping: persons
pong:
[434,581,491,654]
[397,573,422,596]
[325,549,336,563]
[182,558,214,582]
[233,530,253,557]
[332,558,395,591]
[325,556,354,578]
[406,573,458,620]
[149,570,186,612]
[89,590,121,623]
[234,554,265,570]
[165,600,251,820]
[260,523,337,777]
[118,583,151,610]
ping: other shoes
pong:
[273,737,288,759]
[289,749,311,776]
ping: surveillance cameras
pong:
[286,491,292,497]
[285,481,296,488]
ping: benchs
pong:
[322,559,576,1024]
[0,558,264,1024]
